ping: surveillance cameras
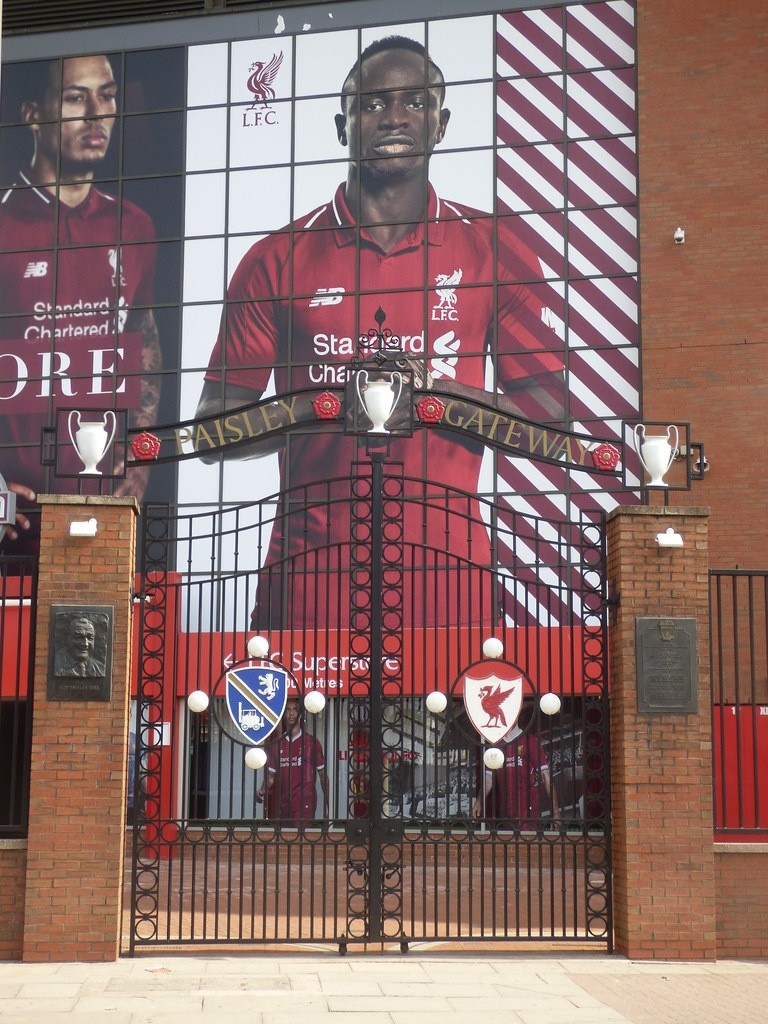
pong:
[674,230,685,242]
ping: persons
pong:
[56,618,105,676]
[189,33,574,631]
[470,715,563,831]
[0,50,164,572]
[255,698,329,826]
